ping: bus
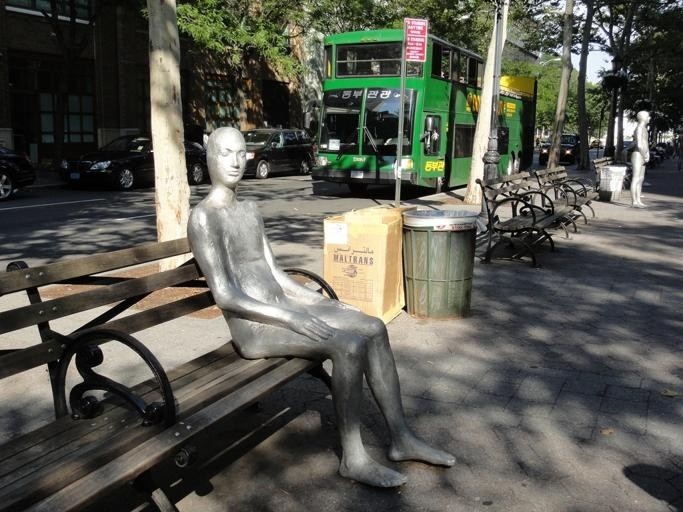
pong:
[312,29,538,194]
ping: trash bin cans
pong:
[599,166,627,203]
[401,210,479,321]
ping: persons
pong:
[185,123,457,489]
[630,109,653,208]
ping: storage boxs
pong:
[322,205,417,326]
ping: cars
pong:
[57,129,317,192]
[539,133,578,165]
[590,140,603,149]
[614,141,666,168]
[0,145,38,200]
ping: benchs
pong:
[0,237,341,512]
[606,156,632,189]
[592,157,633,194]
[534,168,595,224]
[475,170,576,267]
